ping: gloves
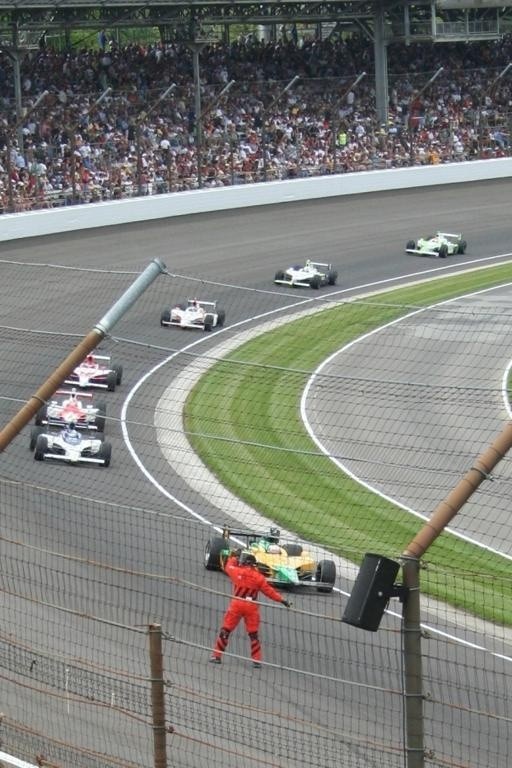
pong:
[282,599,293,608]
[234,549,242,557]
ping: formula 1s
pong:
[203,523,336,593]
[404,230,467,258]
[273,258,339,289]
[29,349,123,467]
[160,296,226,331]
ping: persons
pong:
[0,1,512,218]
[208,551,291,668]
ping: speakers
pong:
[341,553,400,632]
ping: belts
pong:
[234,595,257,601]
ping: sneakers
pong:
[208,657,221,664]
[253,662,262,668]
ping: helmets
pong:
[265,527,280,543]
[238,552,256,567]
